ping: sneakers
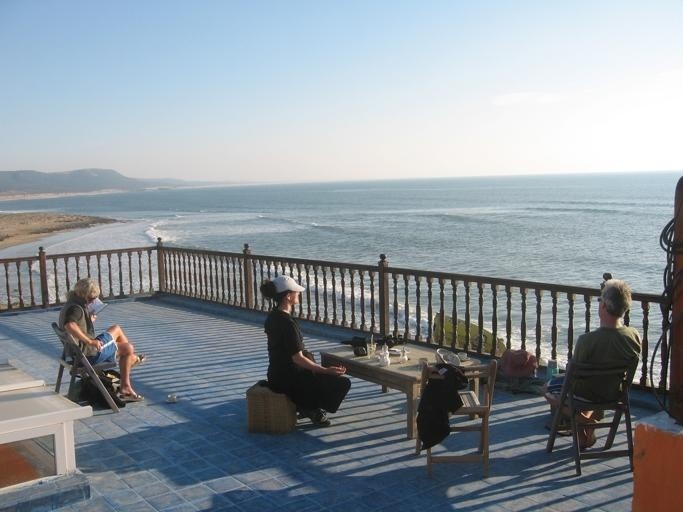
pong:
[296,402,331,427]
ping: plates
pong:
[386,346,411,354]
[457,357,469,361]
[164,399,179,404]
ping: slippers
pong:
[118,390,144,403]
[543,417,574,437]
[129,351,146,369]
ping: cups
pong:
[366,342,408,368]
[167,394,176,402]
[457,352,467,360]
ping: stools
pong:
[246,380,297,435]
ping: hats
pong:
[433,346,463,368]
[273,274,307,295]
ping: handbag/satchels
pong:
[501,348,539,380]
[69,369,126,411]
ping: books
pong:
[84,296,107,315]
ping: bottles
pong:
[546,359,557,382]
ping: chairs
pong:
[51,322,120,412]
[416,359,498,479]
[546,359,633,476]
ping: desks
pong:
[321,342,481,439]
[0,347,93,491]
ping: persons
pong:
[260,276,350,428]
[59,278,146,403]
[544,279,640,449]
